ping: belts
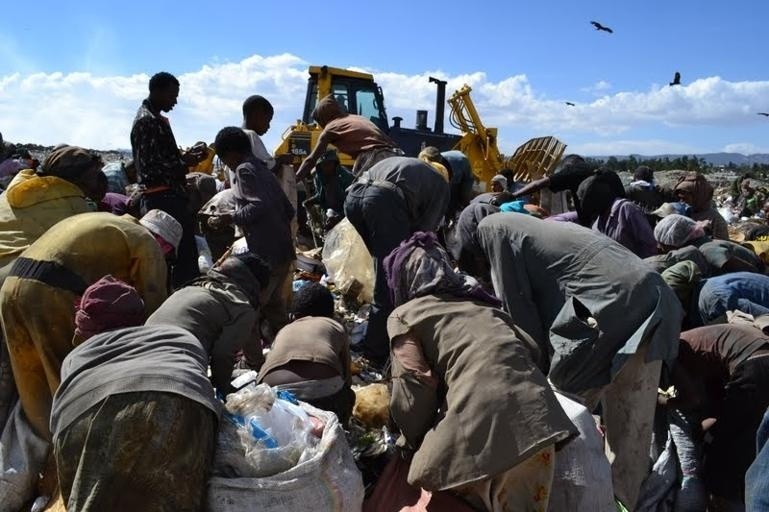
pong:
[358,177,405,199]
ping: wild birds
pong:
[670,72,681,85]
[757,113,769,116]
[590,21,613,33]
[565,101,575,108]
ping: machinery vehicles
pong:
[273,63,569,184]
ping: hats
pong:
[139,208,184,256]
[636,166,650,180]
